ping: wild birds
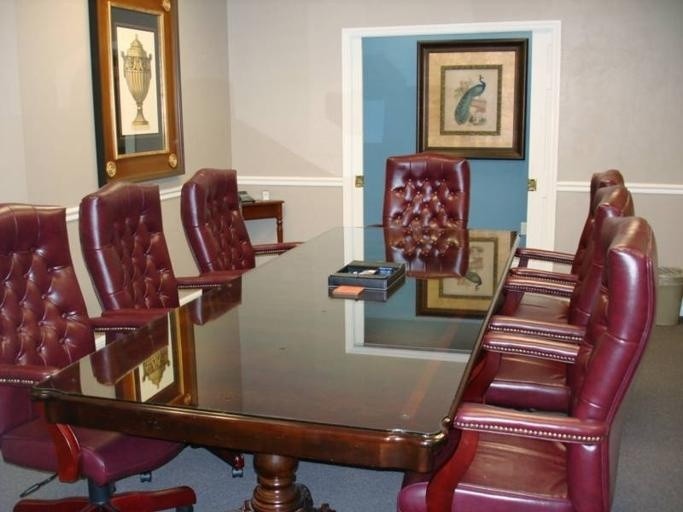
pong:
[454,74,486,125]
[464,272,482,291]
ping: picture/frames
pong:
[415,231,516,319]
[416,38,528,160]
[88,0,186,188]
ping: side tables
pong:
[241,200,285,242]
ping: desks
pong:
[36,224,515,512]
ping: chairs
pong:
[81,181,245,483]
[511,170,623,281]
[385,225,468,278]
[382,153,468,232]
[180,168,306,278]
[487,183,636,342]
[0,204,198,512]
[397,219,659,512]
